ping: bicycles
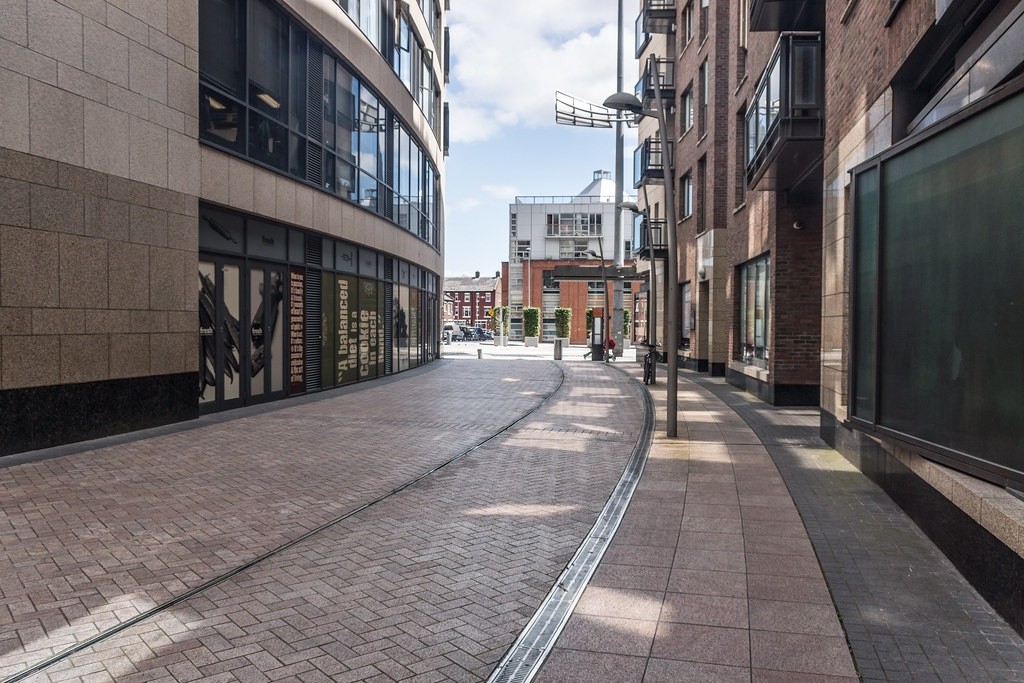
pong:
[640,339,662,385]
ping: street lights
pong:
[602,52,679,439]
[616,179,657,385]
[582,236,610,363]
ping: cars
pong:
[443,322,494,342]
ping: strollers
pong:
[602,339,616,362]
